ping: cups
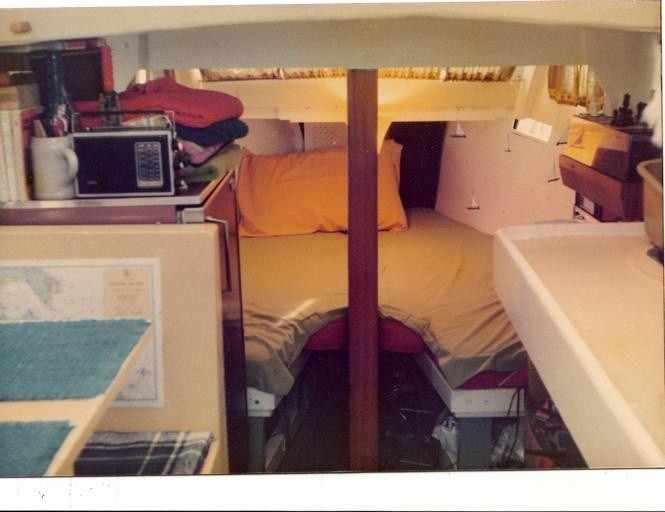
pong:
[29,135,77,199]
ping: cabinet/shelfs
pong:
[0,147,260,477]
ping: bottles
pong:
[42,56,72,137]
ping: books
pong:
[2,114,20,200]
[0,112,10,203]
[10,107,43,202]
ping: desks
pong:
[485,210,663,469]
[0,314,160,479]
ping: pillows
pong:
[226,137,410,238]
[225,116,305,157]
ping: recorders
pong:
[71,109,177,198]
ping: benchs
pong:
[215,115,535,472]
